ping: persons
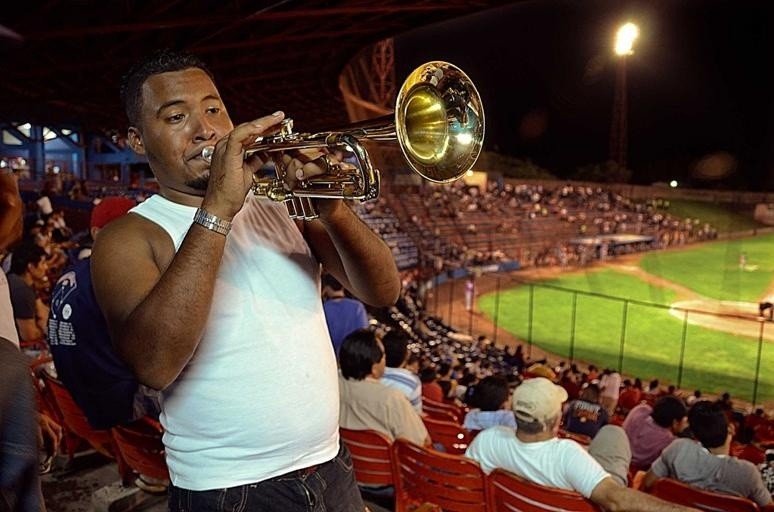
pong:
[345,199,400,259]
[724,407,773,464]
[405,328,465,410]
[507,344,557,399]
[322,274,370,358]
[638,400,774,511]
[1,167,62,508]
[557,360,621,438]
[465,335,507,377]
[719,392,732,410]
[465,376,704,511]
[411,175,717,268]
[620,395,689,469]
[370,276,449,328]
[465,272,477,311]
[461,375,519,430]
[461,375,486,407]
[87,53,402,511]
[337,329,453,512]
[3,173,156,473]
[616,378,703,412]
[46,197,165,492]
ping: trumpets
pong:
[203,61,486,221]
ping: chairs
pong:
[20,339,769,512]
[347,193,607,274]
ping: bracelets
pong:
[193,208,233,235]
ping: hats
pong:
[90,196,136,228]
[511,378,568,423]
